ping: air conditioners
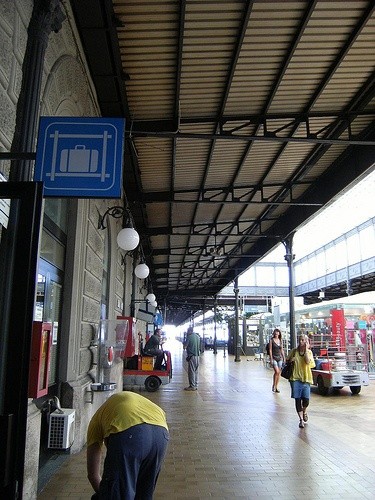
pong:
[47,409,76,449]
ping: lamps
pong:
[210,248,225,257]
[100,206,139,251]
[133,287,158,308]
[122,251,149,279]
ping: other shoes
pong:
[195,387,198,390]
[276,389,280,393]
[272,388,275,392]
[184,386,195,391]
[299,422,305,428]
[154,366,163,370]
[303,413,308,422]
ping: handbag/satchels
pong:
[186,356,192,361]
[280,363,292,379]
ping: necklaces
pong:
[298,348,305,356]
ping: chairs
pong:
[138,333,154,356]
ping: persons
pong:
[269,330,284,393]
[144,329,166,368]
[86,392,169,500]
[183,327,200,391]
[285,334,316,428]
